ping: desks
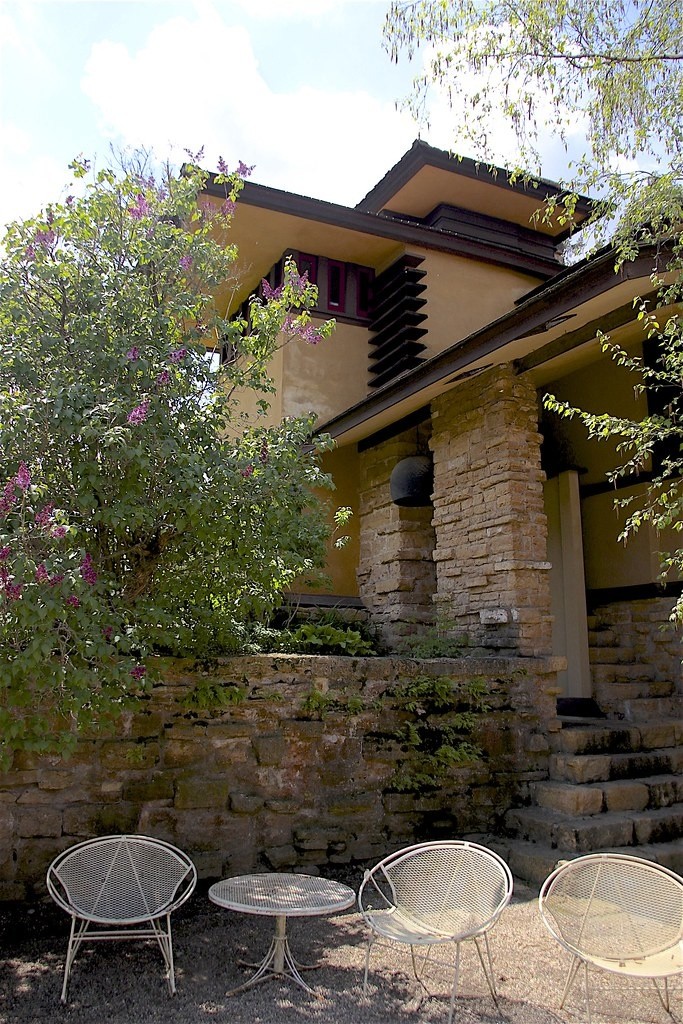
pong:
[209,872,357,1003]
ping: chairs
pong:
[359,840,513,1024]
[537,853,683,1024]
[46,835,198,1005]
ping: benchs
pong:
[278,591,367,610]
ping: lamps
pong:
[390,425,436,507]
